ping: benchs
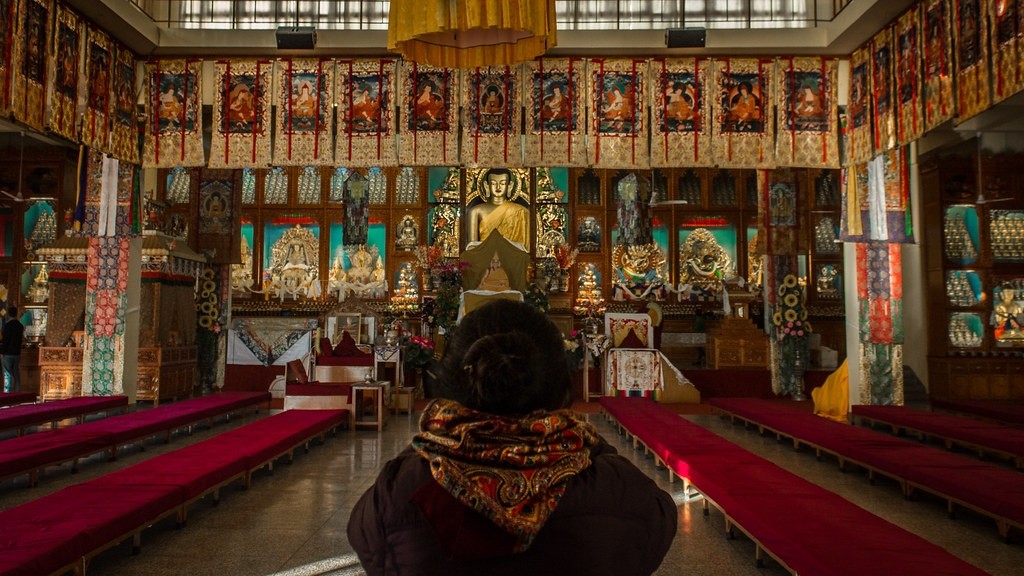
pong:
[0,409,350,576]
[0,391,273,488]
[0,392,37,408]
[596,395,993,576]
[709,396,1024,544]
[927,393,1024,429]
[851,404,1024,471]
[0,395,129,437]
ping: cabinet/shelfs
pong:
[920,156,1024,400]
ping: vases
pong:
[422,275,433,292]
[793,342,808,401]
[414,368,425,401]
[557,272,570,292]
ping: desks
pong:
[374,346,405,415]
[350,381,391,432]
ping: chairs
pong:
[284,359,364,422]
[317,352,374,382]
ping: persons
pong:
[347,300,677,576]
[231,168,1024,346]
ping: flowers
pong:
[195,268,222,334]
[434,262,473,340]
[403,336,437,374]
[772,274,813,339]
[553,242,579,274]
[412,245,443,275]
[523,280,551,316]
[563,330,583,371]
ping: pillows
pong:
[320,337,333,356]
[333,332,364,356]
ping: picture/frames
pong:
[334,312,361,343]
